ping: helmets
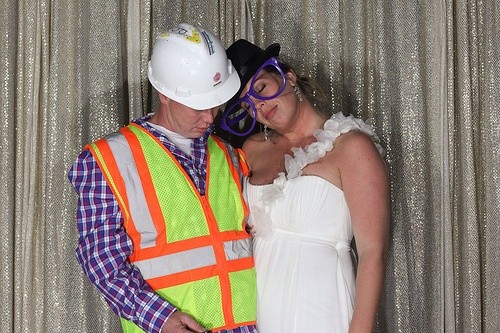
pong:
[147,23,241,111]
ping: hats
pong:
[222,39,280,115]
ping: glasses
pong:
[221,58,287,136]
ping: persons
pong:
[226,39,389,333]
[68,23,258,333]
